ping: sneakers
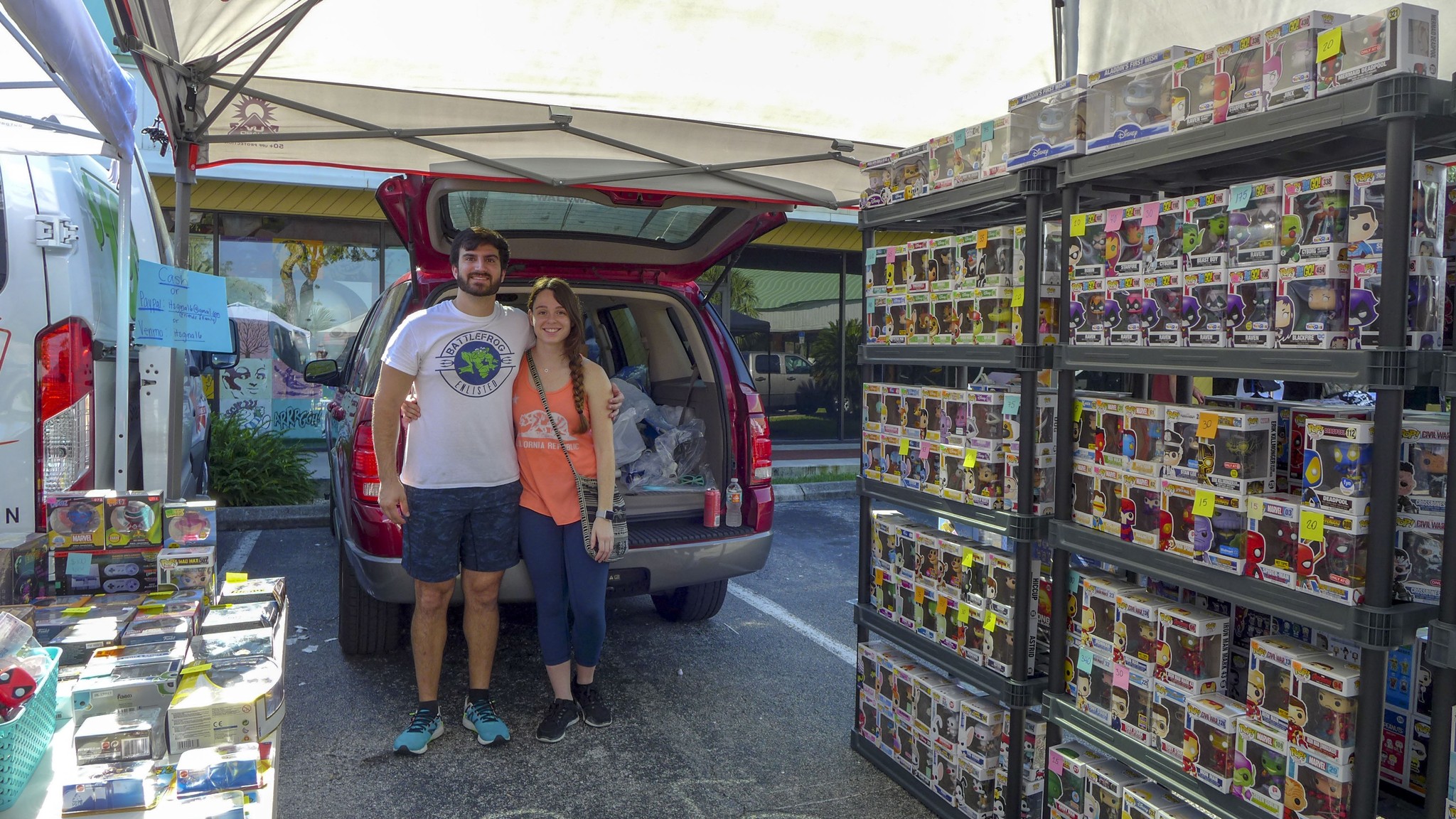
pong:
[536,696,579,742]
[462,695,510,745]
[393,704,444,755]
[570,674,612,727]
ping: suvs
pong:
[300,161,788,659]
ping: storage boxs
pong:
[850,4,1455,819]
[0,489,286,819]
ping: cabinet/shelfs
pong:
[852,205,1040,818]
[1036,74,1454,819]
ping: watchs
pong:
[595,510,615,521]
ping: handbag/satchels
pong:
[576,475,628,563]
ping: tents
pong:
[115,0,1456,501]
[0,0,138,495]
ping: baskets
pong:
[0,647,63,811]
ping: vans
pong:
[741,351,853,420]
[0,150,245,563]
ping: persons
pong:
[372,225,625,755]
[400,276,614,742]
